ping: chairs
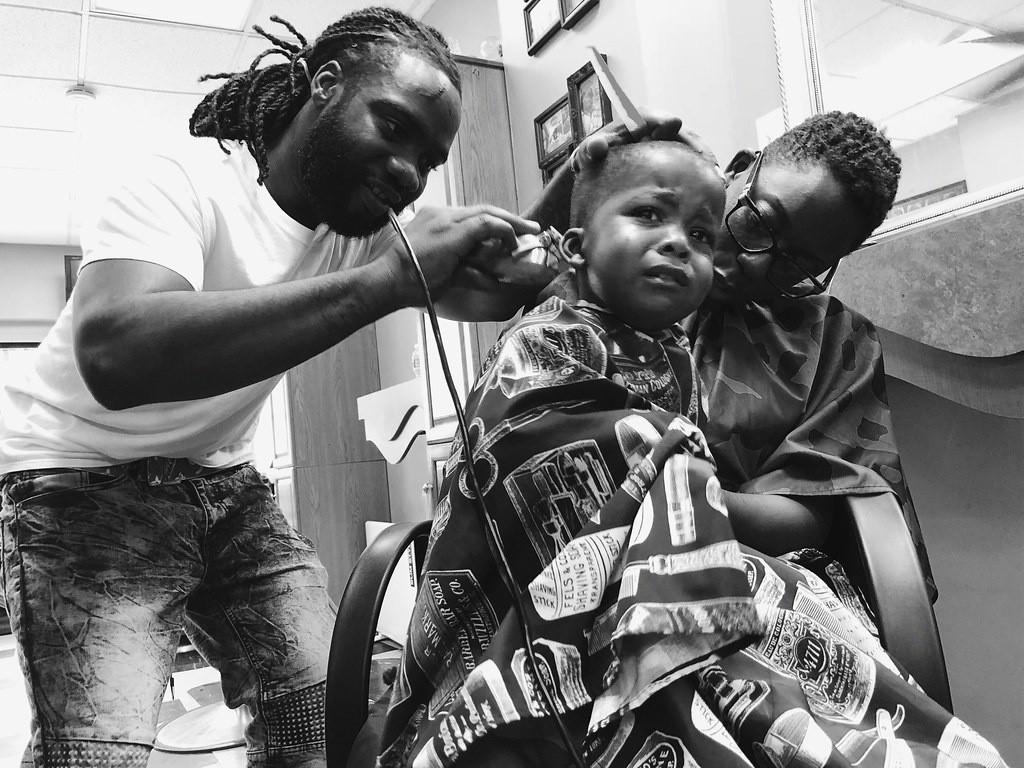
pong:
[326,463,955,768]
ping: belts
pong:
[73,456,221,487]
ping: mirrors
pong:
[770,0,1024,240]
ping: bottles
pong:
[411,344,421,379]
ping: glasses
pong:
[726,150,839,299]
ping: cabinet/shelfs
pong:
[374,53,525,525]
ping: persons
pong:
[406,111,941,768]
[0,7,542,768]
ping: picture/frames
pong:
[523,0,599,57]
[542,153,571,190]
[534,92,576,170]
[567,52,613,149]
